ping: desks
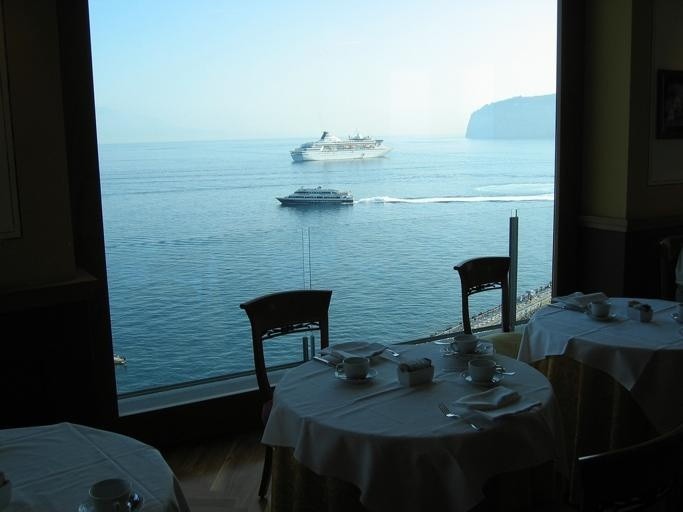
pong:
[0,271,106,419]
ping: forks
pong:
[439,401,480,432]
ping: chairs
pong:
[577,424,683,511]
[659,234,683,300]
[241,291,334,500]
[454,256,512,335]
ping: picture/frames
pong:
[656,69,683,139]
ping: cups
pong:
[450,334,480,353]
[335,356,370,379]
[89,478,134,512]
[587,301,612,318]
[467,358,503,385]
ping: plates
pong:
[78,490,144,512]
[461,370,504,388]
[587,311,617,321]
[334,367,378,384]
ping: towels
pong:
[552,291,608,309]
[453,385,541,420]
[316,341,387,361]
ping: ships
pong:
[288,130,388,160]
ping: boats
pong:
[276,186,353,207]
